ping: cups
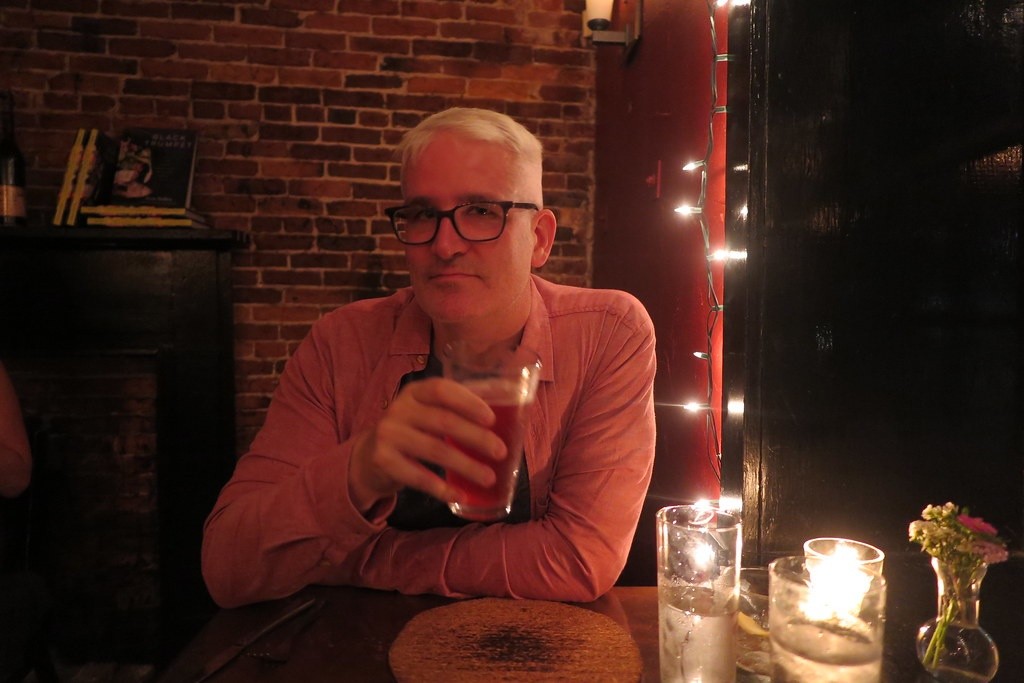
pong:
[804,537,883,577]
[437,334,541,519]
[651,501,744,683]
[733,561,784,683]
[765,557,887,683]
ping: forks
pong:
[244,600,327,664]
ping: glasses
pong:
[385,201,538,246]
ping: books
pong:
[0,126,212,228]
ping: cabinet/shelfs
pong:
[0,218,238,683]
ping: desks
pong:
[161,541,1024,683]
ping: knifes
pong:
[190,596,318,683]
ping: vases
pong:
[916,557,999,683]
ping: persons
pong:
[200,107,657,607]
[0,357,34,496]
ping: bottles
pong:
[0,91,27,227]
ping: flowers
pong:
[907,502,1010,565]
